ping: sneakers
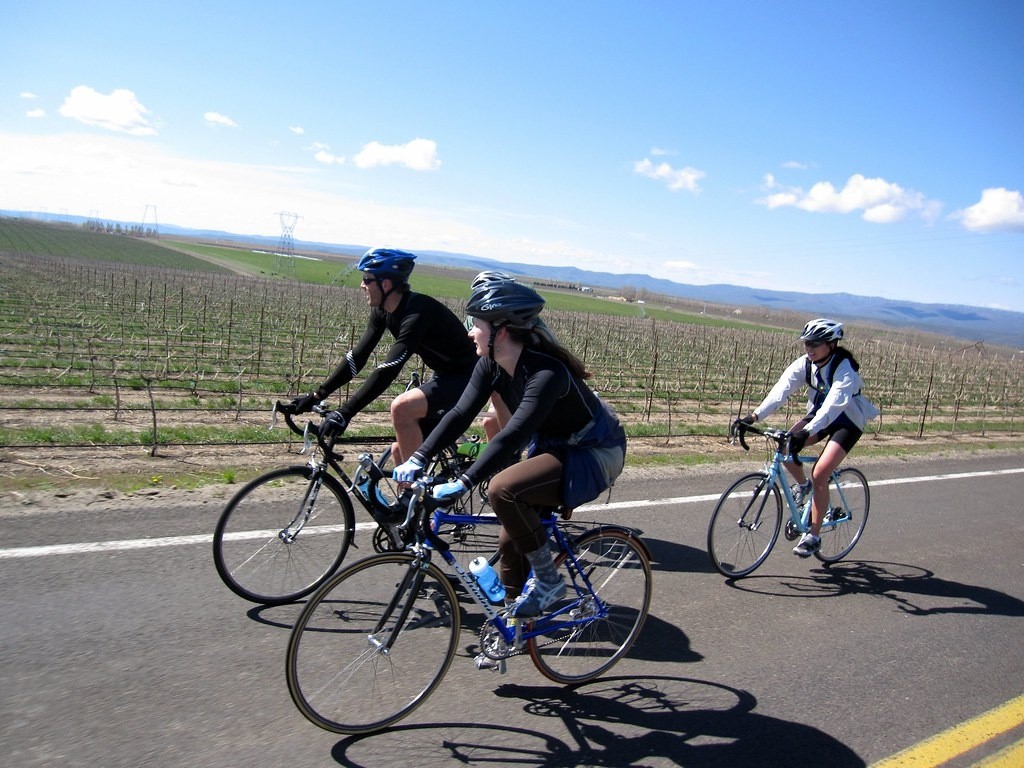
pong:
[473,643,513,669]
[792,533,821,559]
[786,477,814,509]
[509,573,569,617]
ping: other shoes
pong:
[390,487,415,507]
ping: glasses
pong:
[360,276,381,285]
[803,340,825,348]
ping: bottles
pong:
[469,556,507,602]
[357,475,389,508]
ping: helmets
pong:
[800,318,844,341]
[357,247,418,283]
[464,282,546,329]
[470,270,516,291]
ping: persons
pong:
[393,271,630,617]
[741,318,879,558]
[288,246,478,518]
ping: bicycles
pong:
[706,417,874,582]
[212,399,506,609]
[284,445,654,735]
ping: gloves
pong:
[789,430,809,453]
[289,393,320,415]
[731,415,754,435]
[318,409,351,439]
